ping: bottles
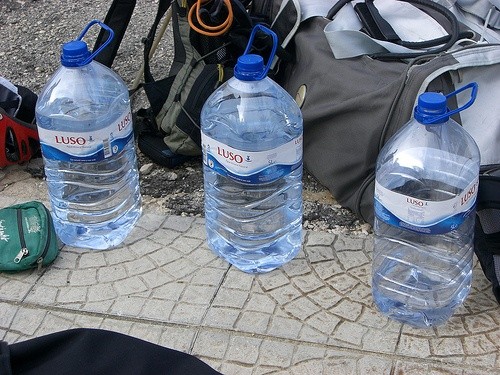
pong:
[35,21,142,249]
[373,82,481,328]
[200,25,303,274]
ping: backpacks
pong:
[92,0,302,167]
[268,0,500,230]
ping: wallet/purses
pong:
[0,201,60,275]
